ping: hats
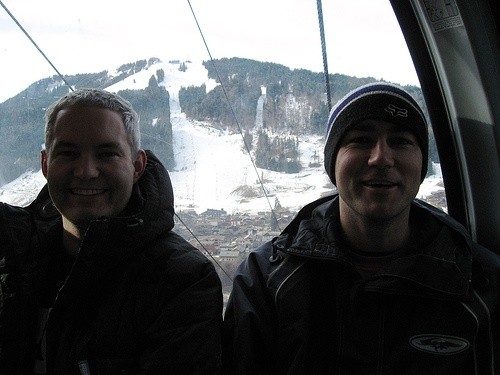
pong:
[324,82,429,187]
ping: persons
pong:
[223,83,500,375]
[0,88,223,374]
